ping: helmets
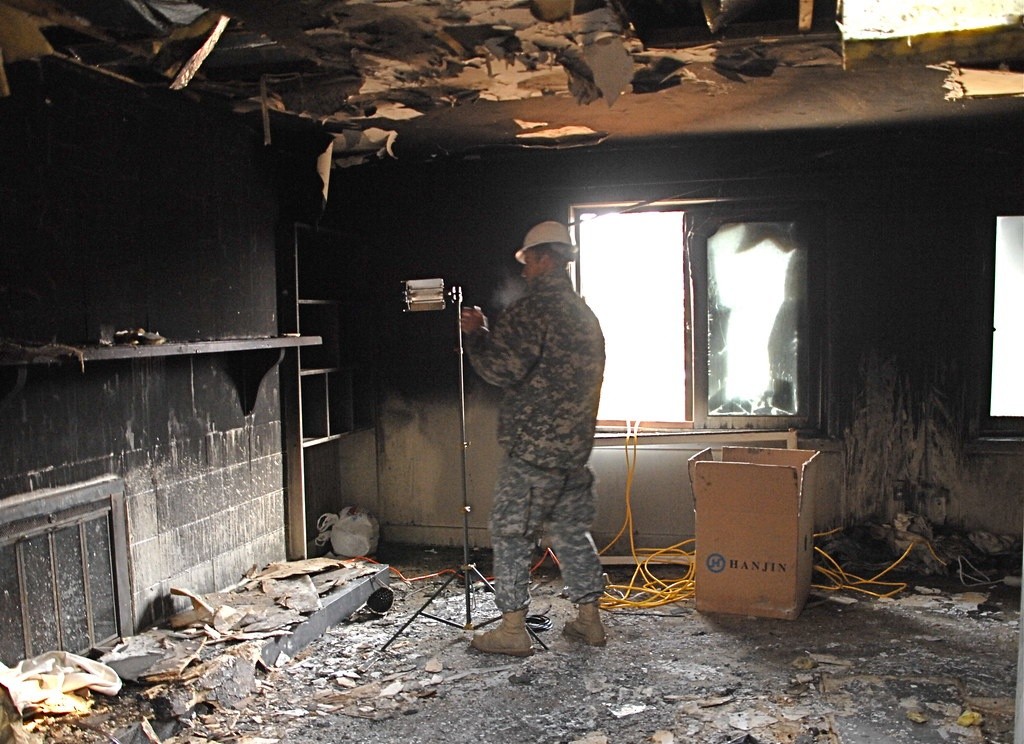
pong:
[515,221,572,264]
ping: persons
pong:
[459,220,608,657]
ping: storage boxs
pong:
[687,446,820,621]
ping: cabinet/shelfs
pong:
[278,223,380,561]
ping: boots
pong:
[564,602,606,647]
[471,606,532,656]
